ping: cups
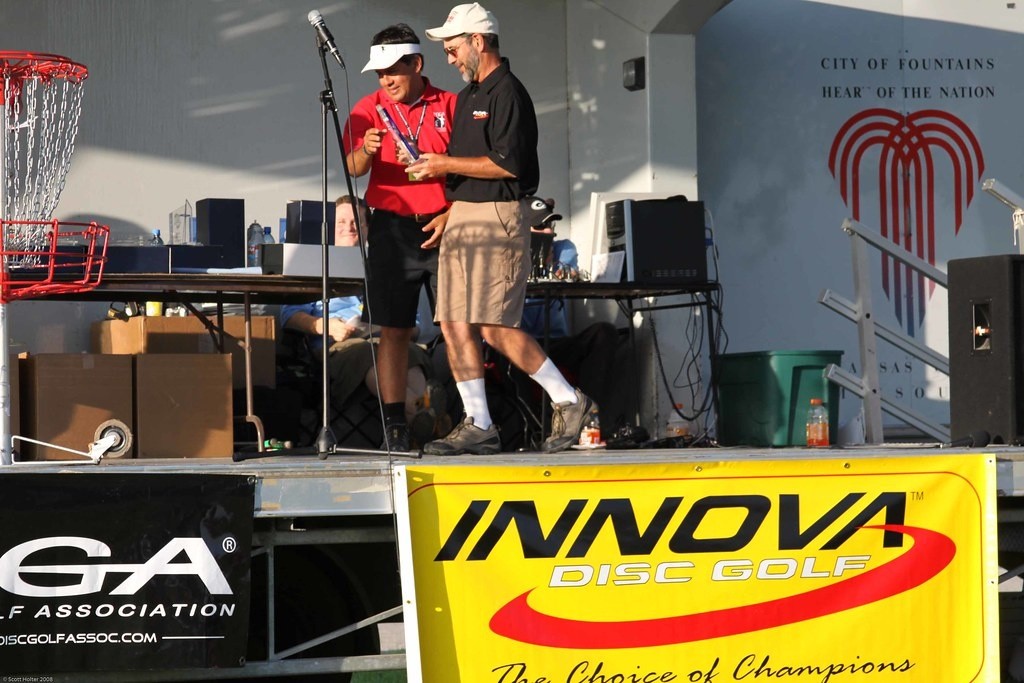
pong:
[146,302,163,316]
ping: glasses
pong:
[444,37,472,58]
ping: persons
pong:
[280,194,437,451]
[482,195,618,451]
[405,3,599,456]
[343,23,457,456]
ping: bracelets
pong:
[364,145,374,156]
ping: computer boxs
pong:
[605,200,708,284]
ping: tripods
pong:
[232,45,423,462]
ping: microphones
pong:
[939,429,992,450]
[307,9,346,70]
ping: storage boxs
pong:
[9,315,277,460]
[282,243,367,278]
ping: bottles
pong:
[579,410,600,446]
[150,230,164,246]
[666,403,690,436]
[263,227,276,244]
[807,399,830,447]
[247,218,263,267]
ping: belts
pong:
[374,208,446,222]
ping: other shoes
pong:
[409,410,437,443]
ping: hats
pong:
[424,2,499,41]
[526,196,563,227]
[360,43,423,73]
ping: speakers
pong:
[196,198,245,268]
[947,254,1024,446]
[285,200,335,244]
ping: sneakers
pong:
[379,423,410,452]
[543,387,595,453]
[424,417,501,456]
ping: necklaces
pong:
[394,102,427,143]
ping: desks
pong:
[8,273,366,453]
[486,282,721,453]
